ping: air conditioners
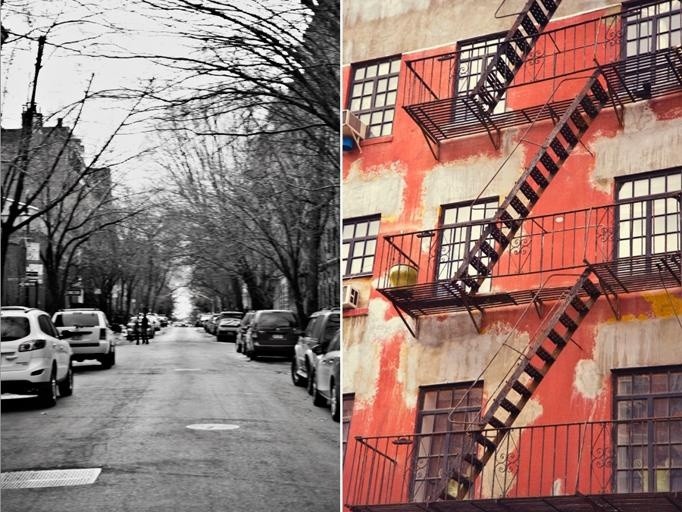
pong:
[343,111,366,139]
[344,286,359,308]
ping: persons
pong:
[133,314,148,345]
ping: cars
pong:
[127,311,169,340]
[196,309,340,420]
[0,306,115,406]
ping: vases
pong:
[389,264,418,286]
